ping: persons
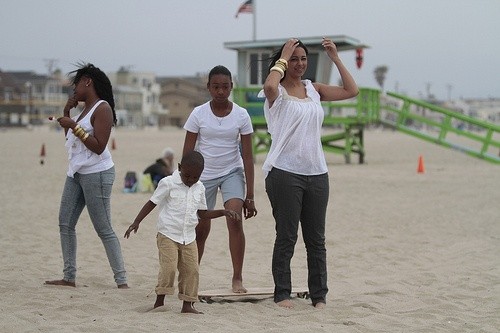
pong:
[123,151,240,314]
[182,65,258,294]
[144,152,174,189]
[44,61,131,290]
[249,38,359,308]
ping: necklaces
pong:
[211,101,229,126]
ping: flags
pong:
[235,0,253,18]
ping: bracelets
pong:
[71,124,89,143]
[246,198,254,203]
[270,59,289,79]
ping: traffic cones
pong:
[416,155,425,174]
[39,144,46,156]
[111,138,116,150]
